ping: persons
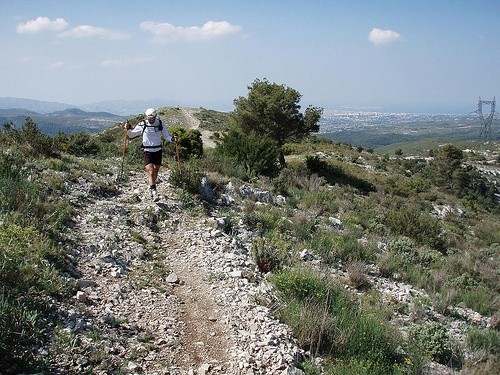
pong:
[124,108,178,201]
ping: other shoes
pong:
[149,188,160,201]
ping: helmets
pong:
[145,108,156,120]
